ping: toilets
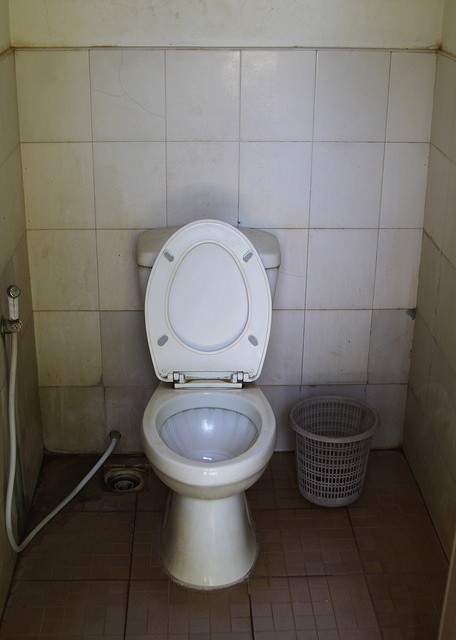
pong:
[136,218,281,591]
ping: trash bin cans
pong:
[289,395,379,508]
